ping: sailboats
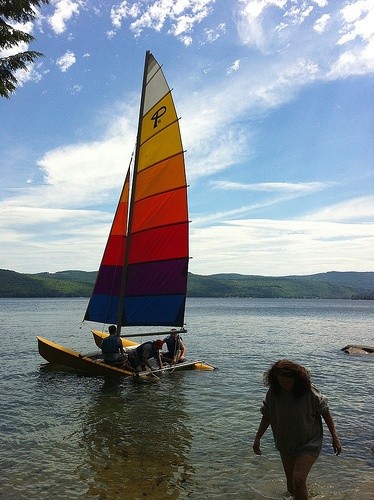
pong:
[36,51,219,379]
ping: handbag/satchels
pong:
[128,349,142,370]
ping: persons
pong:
[163,328,186,365]
[129,339,164,372]
[100,325,129,372]
[252,359,341,500]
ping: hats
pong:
[154,340,163,350]
